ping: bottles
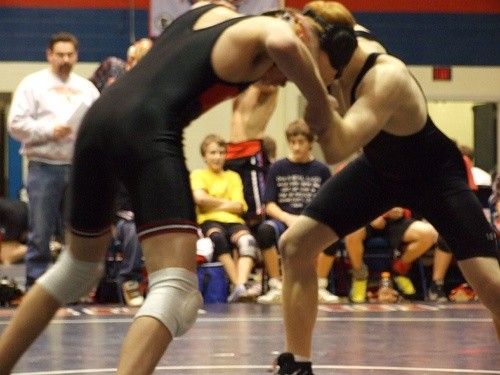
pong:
[378,271,395,303]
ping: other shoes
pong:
[268,352,313,375]
[79,273,479,304]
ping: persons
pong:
[223,80,280,228]
[251,119,343,306]
[213,1,500,374]
[424,146,499,303]
[90,36,156,94]
[0,1,341,375]
[81,211,146,309]
[187,135,260,302]
[6,32,102,297]
[336,144,440,303]
